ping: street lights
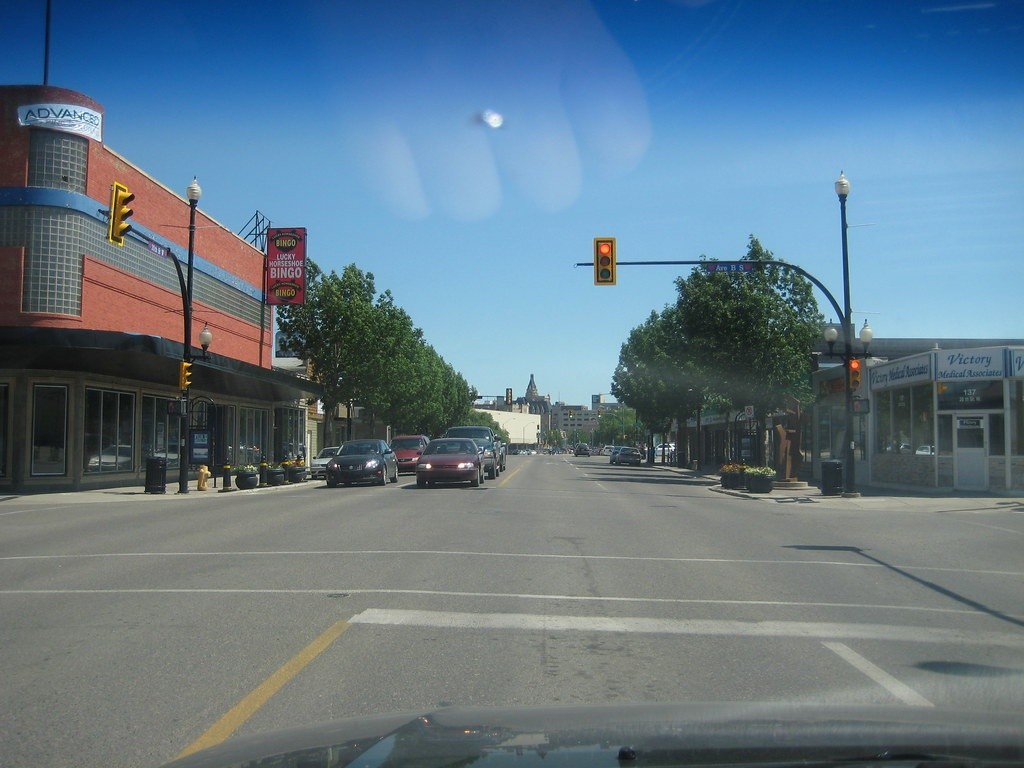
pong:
[825,169,872,493]
[182,177,211,494]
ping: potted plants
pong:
[288,463,306,483]
[233,463,258,489]
[717,461,776,494]
[266,462,287,485]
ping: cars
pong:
[326,439,399,488]
[654,443,676,456]
[389,435,432,472]
[437,426,506,479]
[508,449,572,456]
[310,446,341,479]
[417,439,484,487]
[609,446,641,465]
[573,443,615,457]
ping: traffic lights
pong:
[597,409,601,418]
[569,410,573,418]
[594,237,617,286]
[852,397,870,414]
[850,359,862,390]
[108,184,135,245]
[180,362,192,391]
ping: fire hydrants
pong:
[196,465,211,493]
